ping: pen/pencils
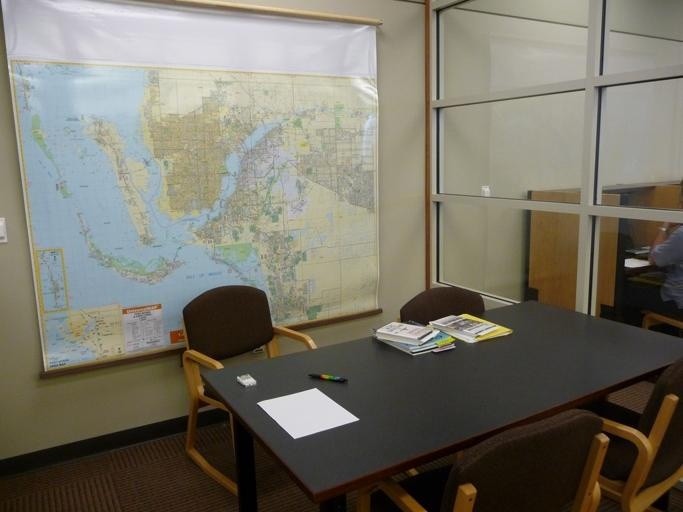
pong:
[308,373,348,383]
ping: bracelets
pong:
[656,226,669,233]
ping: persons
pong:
[625,179,683,327]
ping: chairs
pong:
[354,409,611,512]
[594,359,682,511]
[399,286,485,326]
[181,286,317,498]
[643,312,682,330]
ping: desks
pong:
[198,298,683,512]
[526,183,683,318]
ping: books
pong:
[370,314,514,356]
